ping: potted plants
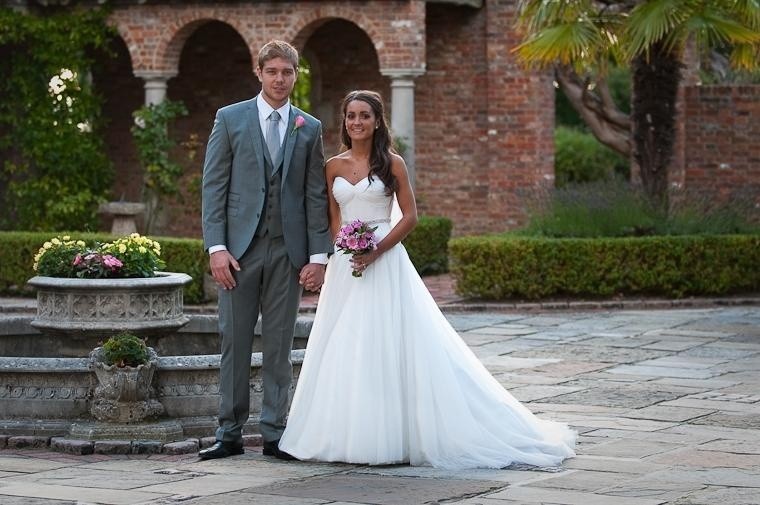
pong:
[89,334,158,401]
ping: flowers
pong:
[334,218,379,278]
[33,233,167,278]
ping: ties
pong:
[267,111,281,168]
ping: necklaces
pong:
[349,158,368,179]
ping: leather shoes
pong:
[262,440,295,461]
[198,441,243,460]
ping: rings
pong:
[362,263,366,266]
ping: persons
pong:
[276,90,432,474]
[198,40,336,464]
